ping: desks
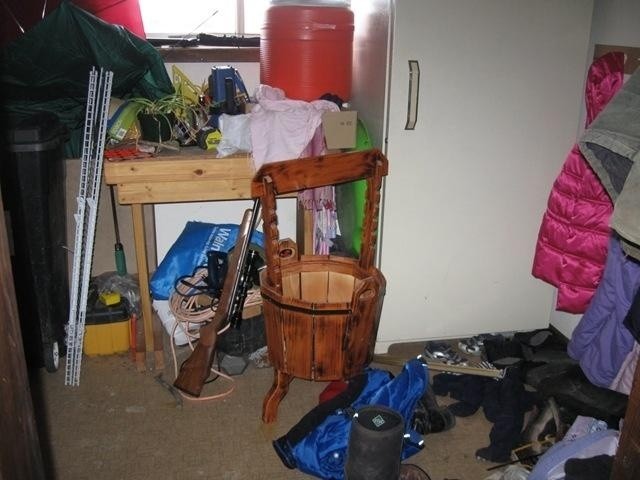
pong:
[104,142,312,350]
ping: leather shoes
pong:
[412,383,455,436]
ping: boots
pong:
[475,396,524,463]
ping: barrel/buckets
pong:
[252,150,388,380]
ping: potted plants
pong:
[139,94,206,144]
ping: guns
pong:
[144,33,262,46]
[173,195,260,397]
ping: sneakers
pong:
[459,334,493,355]
[426,343,470,367]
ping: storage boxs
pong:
[83,296,131,359]
